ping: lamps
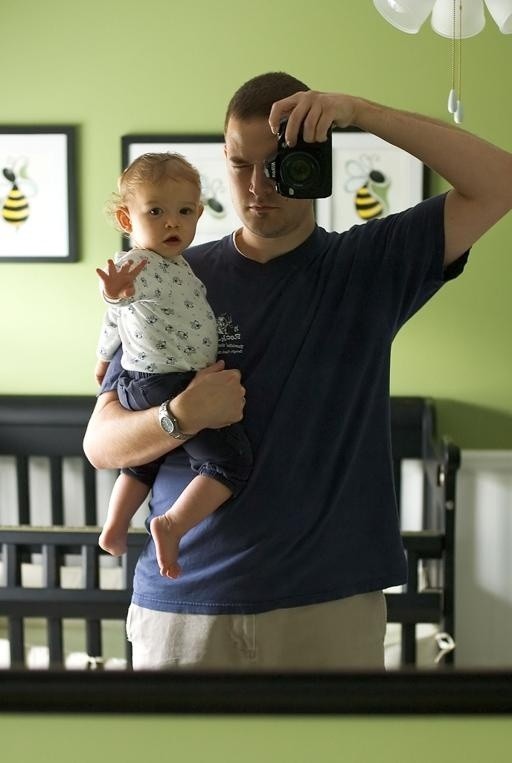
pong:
[372,0,512,123]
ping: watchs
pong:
[158,400,193,442]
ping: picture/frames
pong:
[312,125,430,235]
[119,131,244,256]
[0,124,79,263]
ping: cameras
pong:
[263,120,333,200]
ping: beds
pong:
[0,394,460,673]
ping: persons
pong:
[93,147,256,581]
[78,70,512,674]
